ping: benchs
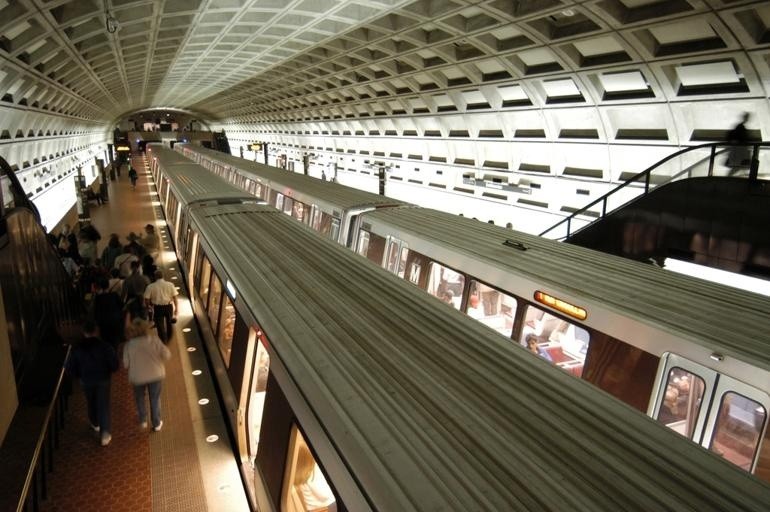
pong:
[49,161,112,240]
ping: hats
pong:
[143,224,154,233]
[125,232,139,240]
[124,317,155,337]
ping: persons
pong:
[442,289,456,306]
[526,332,554,362]
[677,378,691,406]
[126,161,132,177]
[466,294,485,319]
[47,223,180,447]
[657,391,685,425]
[128,166,138,192]
[86,187,108,207]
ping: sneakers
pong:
[90,424,100,433]
[100,434,112,446]
[139,422,148,429]
[154,420,163,432]
